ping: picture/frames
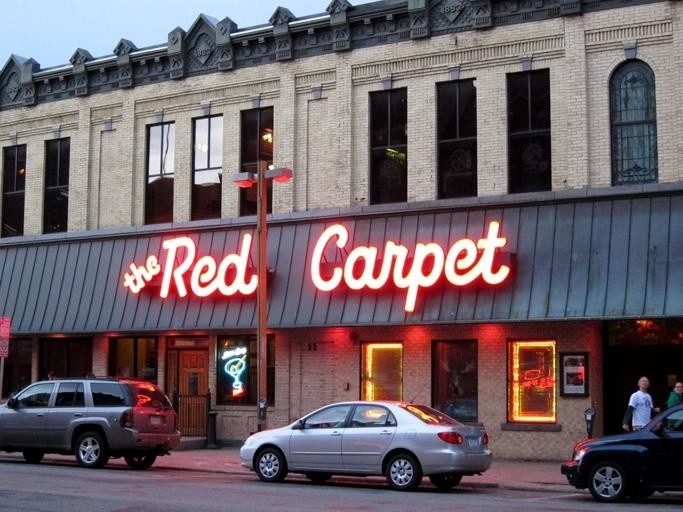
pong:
[559,352,590,398]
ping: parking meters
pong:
[584,408,596,438]
[257,398,267,432]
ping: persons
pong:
[622,376,661,432]
[85,372,95,378]
[48,372,58,380]
[667,382,682,429]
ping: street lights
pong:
[232,160,292,433]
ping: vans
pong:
[0,375,181,471]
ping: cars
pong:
[238,400,494,491]
[560,402,682,502]
